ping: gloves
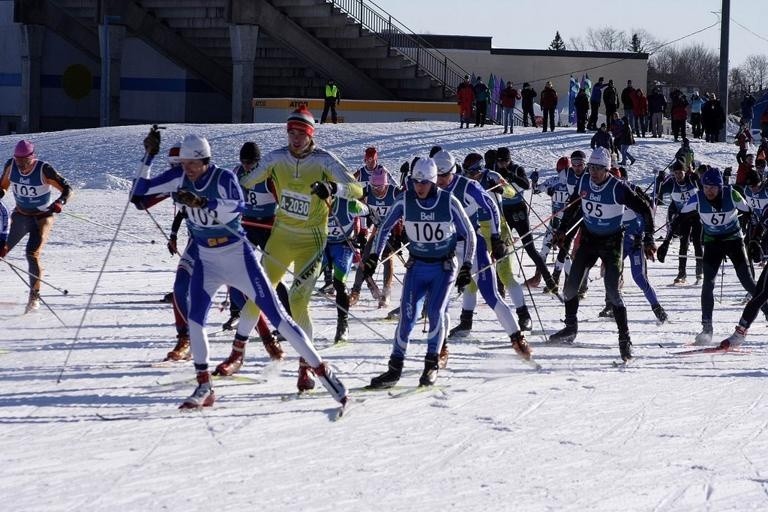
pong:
[642,233,657,262]
[456,266,473,293]
[143,130,161,155]
[47,200,64,213]
[173,188,206,208]
[167,237,177,255]
[363,254,378,273]
[310,181,337,200]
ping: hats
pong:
[556,147,612,172]
[240,142,260,164]
[743,169,761,184]
[286,104,315,138]
[370,165,388,186]
[672,160,686,171]
[401,145,511,184]
[167,142,183,165]
[179,135,211,160]
[701,169,723,186]
[366,146,378,161]
[11,140,35,158]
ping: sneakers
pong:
[516,305,533,331]
[508,332,533,362]
[26,295,40,311]
[449,320,472,338]
[297,363,316,392]
[717,324,752,352]
[548,326,577,344]
[262,337,284,360]
[577,126,664,138]
[371,367,400,389]
[694,322,713,347]
[223,316,239,330]
[178,384,214,411]
[311,363,348,405]
[630,159,636,165]
[271,329,287,343]
[525,272,703,321]
[618,334,632,363]
[166,336,191,362]
[214,350,244,376]
[618,162,626,166]
[460,125,483,128]
[318,281,401,343]
[437,342,449,370]
[420,354,441,387]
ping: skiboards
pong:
[447,272,754,329]
[526,340,612,349]
[613,353,643,367]
[95,394,280,421]
[387,381,451,398]
[331,390,368,422]
[349,383,403,394]
[658,340,762,349]
[152,286,432,392]
[666,343,765,356]
[96,269,767,422]
[409,337,482,345]
[515,351,541,372]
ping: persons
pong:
[0,138,75,315]
[319,78,341,125]
[0,200,13,259]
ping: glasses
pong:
[369,183,386,192]
